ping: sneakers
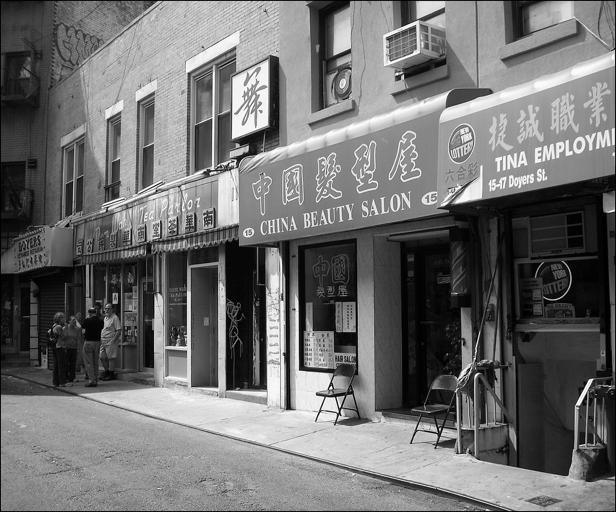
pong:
[55,373,115,388]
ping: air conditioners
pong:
[382,20,447,70]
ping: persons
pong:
[48,300,122,388]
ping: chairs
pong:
[410,374,458,449]
[314,363,361,426]
